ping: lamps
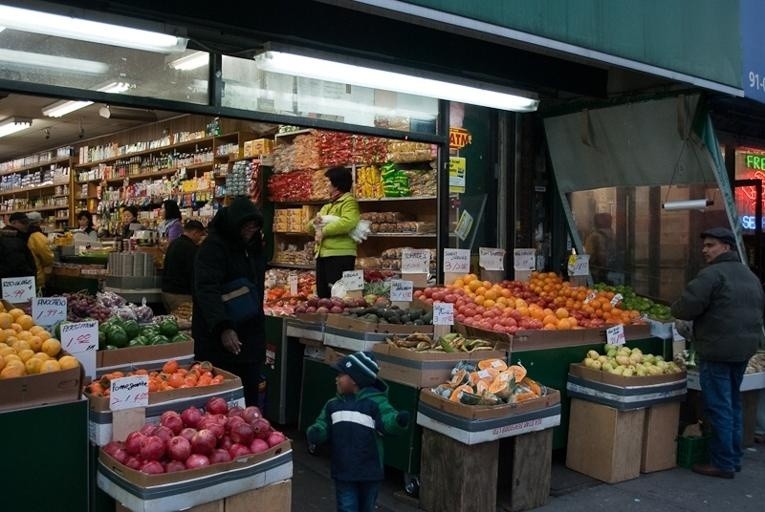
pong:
[0,3,190,56]
[42,99,95,119]
[98,103,158,123]
[0,114,33,140]
[251,39,542,115]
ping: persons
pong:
[305,166,360,300]
[308,351,411,512]
[587,212,615,283]
[25,211,54,298]
[76,210,98,240]
[161,220,204,314]
[191,196,266,409]
[0,212,37,299]
[157,200,184,242]
[115,206,140,252]
[670,227,765,479]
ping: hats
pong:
[330,351,380,388]
[9,212,27,222]
[26,212,43,223]
[699,227,736,246]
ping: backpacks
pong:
[596,230,615,270]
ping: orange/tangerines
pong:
[86,357,230,402]
[528,269,641,325]
[590,282,673,321]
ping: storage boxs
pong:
[564,394,646,484]
[508,427,559,512]
[71,336,197,383]
[84,362,247,450]
[639,399,683,476]
[465,313,654,355]
[568,352,689,409]
[418,429,499,512]
[0,361,81,413]
[96,440,295,512]
[285,305,324,340]
[416,377,563,444]
[372,336,507,391]
[323,303,462,352]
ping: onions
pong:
[107,396,286,473]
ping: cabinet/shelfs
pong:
[0,156,79,244]
[70,130,258,253]
[267,128,444,288]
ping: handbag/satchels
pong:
[217,279,261,327]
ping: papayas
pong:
[432,358,545,408]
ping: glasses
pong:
[243,225,259,232]
[16,220,29,224]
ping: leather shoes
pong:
[733,466,742,473]
[692,465,735,480]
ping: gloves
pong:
[306,428,320,456]
[396,412,410,426]
